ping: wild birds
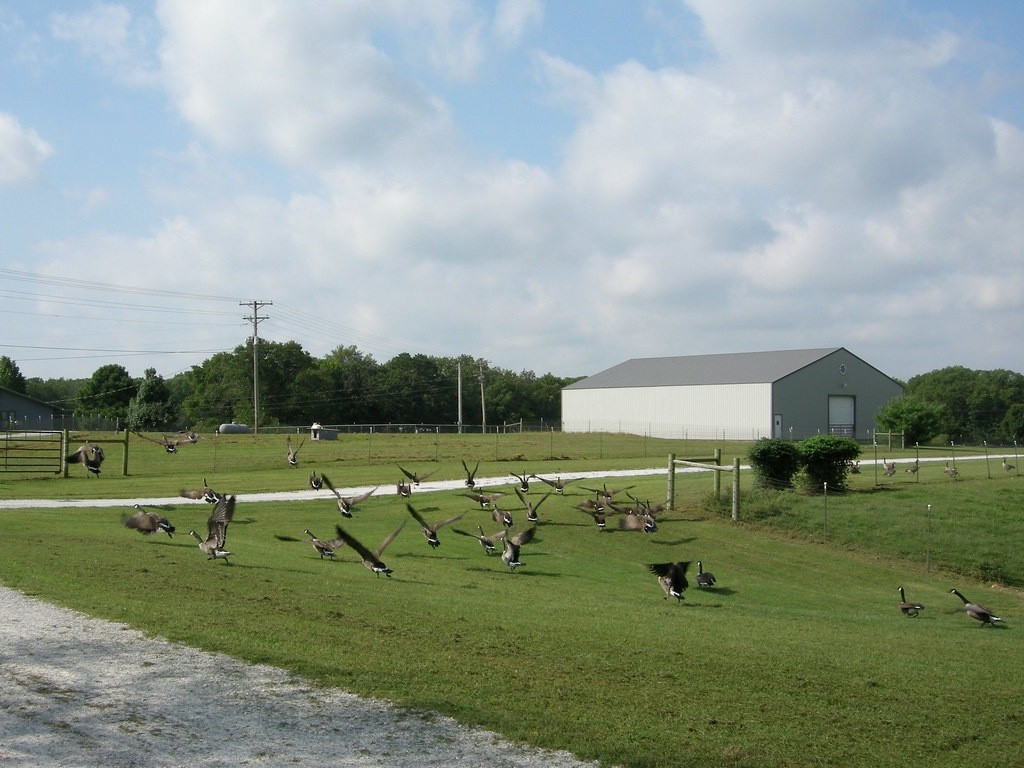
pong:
[696,560,718,590]
[285,435,307,470]
[895,587,926,619]
[120,504,176,539]
[308,470,378,519]
[303,518,409,579]
[849,457,1017,478]
[641,560,694,604]
[179,477,236,565]
[394,459,679,575]
[64,441,105,479]
[162,427,199,455]
[948,589,1008,628]
